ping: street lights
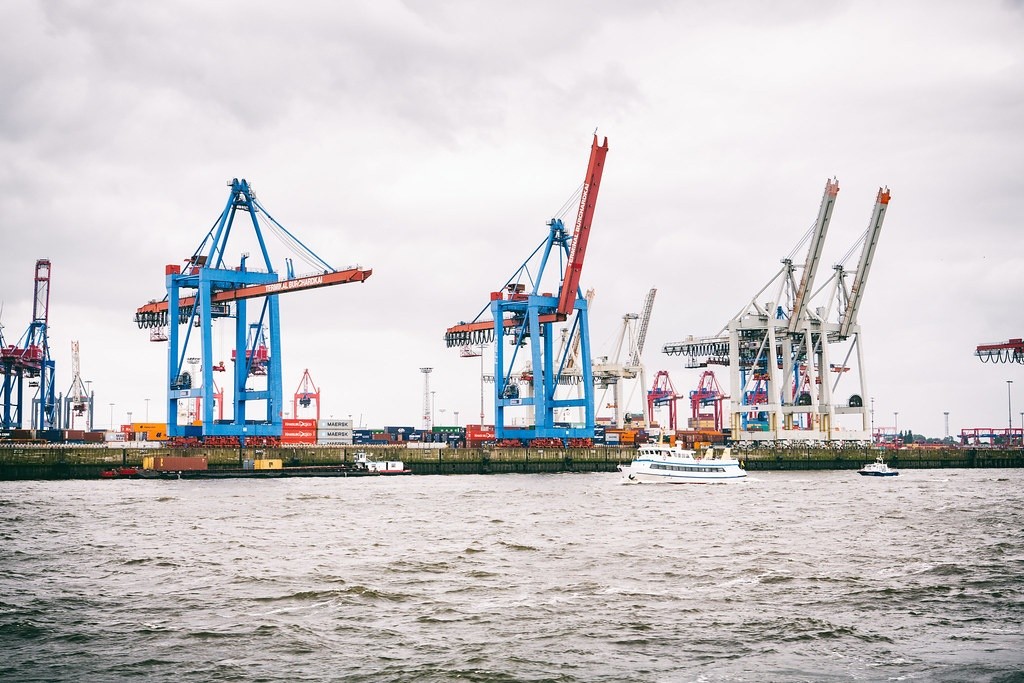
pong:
[893,411,899,439]
[1020,412,1024,446]
[1006,381,1013,444]
[943,411,950,438]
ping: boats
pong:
[345,453,413,477]
[857,450,900,476]
[616,438,749,485]
